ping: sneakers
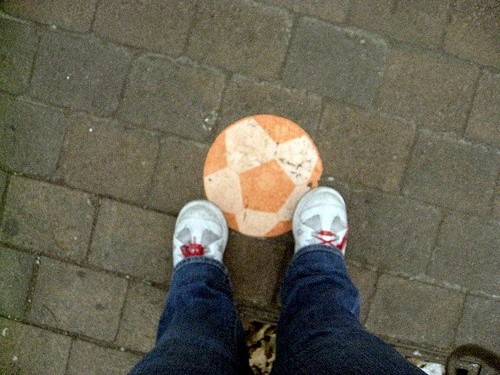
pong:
[291,187,348,257]
[171,199,228,266]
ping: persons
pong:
[126,186,428,375]
